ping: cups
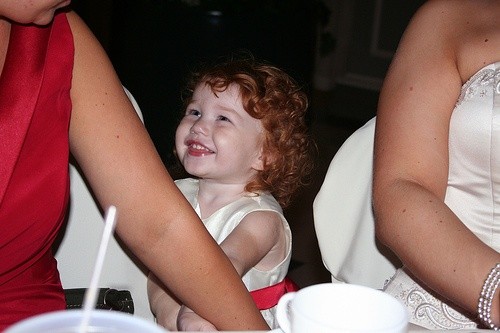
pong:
[2,309,170,333]
[276,283,410,333]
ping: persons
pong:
[0,0,271,333]
[313,2,500,333]
[147,60,315,333]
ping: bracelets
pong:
[478,263,500,332]
